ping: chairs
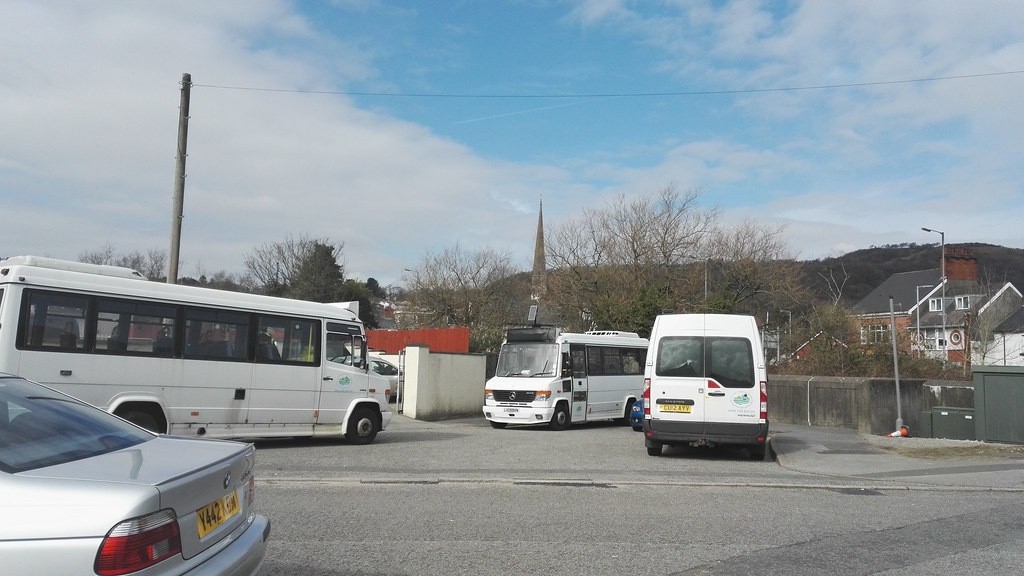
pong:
[59,319,280,362]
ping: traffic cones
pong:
[886,425,910,437]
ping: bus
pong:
[0,256,393,445]
[482,325,648,430]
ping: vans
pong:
[644,314,770,460]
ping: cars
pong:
[629,395,645,432]
[0,371,271,576]
[339,356,403,400]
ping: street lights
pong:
[916,285,933,358]
[921,227,946,362]
[780,310,791,341]
[690,255,708,300]
